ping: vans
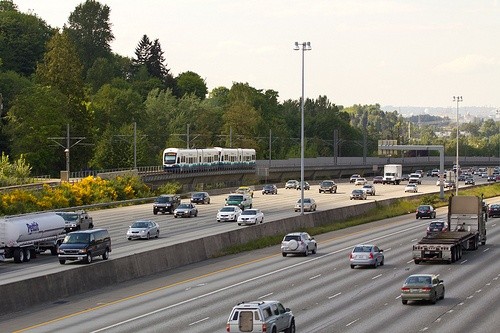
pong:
[57,228,112,265]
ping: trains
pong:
[162,146,257,171]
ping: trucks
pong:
[0,212,67,264]
[382,164,403,185]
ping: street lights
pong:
[452,96,464,195]
[294,41,312,215]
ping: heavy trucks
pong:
[411,192,488,264]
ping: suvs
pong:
[226,300,295,333]
[355,178,367,186]
[361,184,375,196]
[59,211,92,232]
[348,244,385,269]
[318,180,337,194]
[152,194,181,214]
[224,193,252,211]
[349,174,361,183]
[281,232,317,257]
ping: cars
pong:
[191,192,210,204]
[349,189,367,200]
[237,208,264,225]
[126,220,160,240]
[401,273,445,305]
[416,204,437,219]
[402,166,500,193]
[261,184,277,195]
[487,203,500,217]
[284,180,299,189]
[373,176,383,184]
[294,197,317,212]
[296,182,310,190]
[235,187,254,198]
[216,206,242,222]
[173,203,198,218]
